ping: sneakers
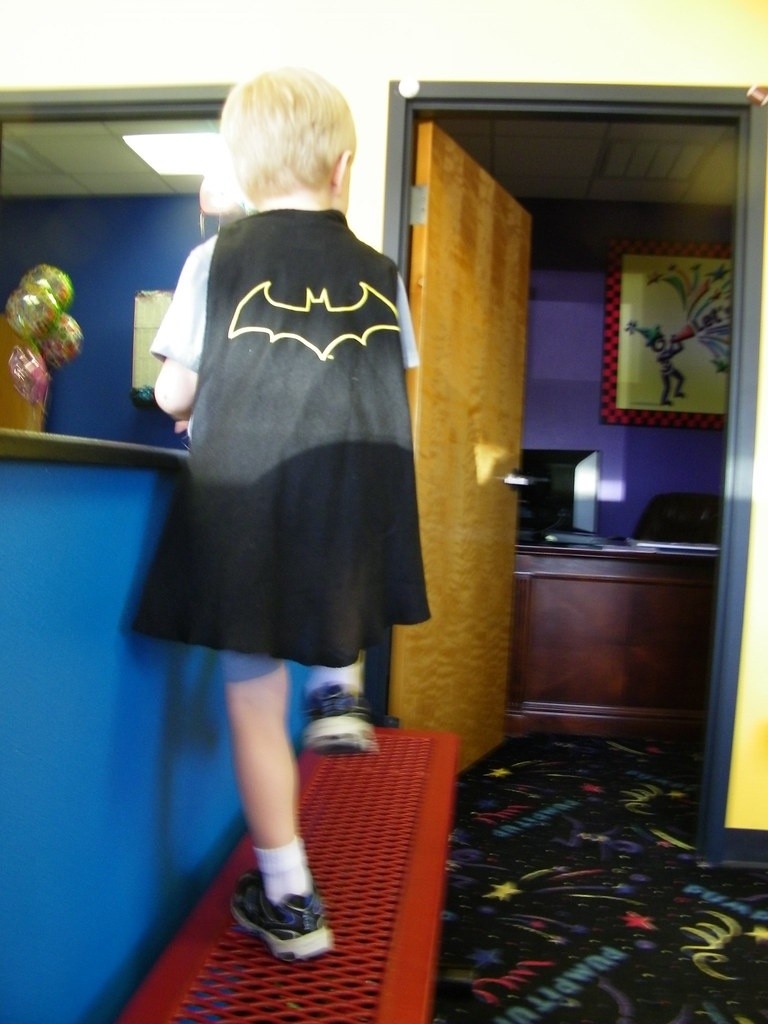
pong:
[303,683,399,756]
[229,867,335,961]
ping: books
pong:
[628,537,718,551]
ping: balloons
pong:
[5,264,81,407]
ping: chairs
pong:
[633,492,721,548]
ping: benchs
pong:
[118,730,462,1024]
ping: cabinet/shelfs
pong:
[503,542,720,739]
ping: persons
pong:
[129,69,431,962]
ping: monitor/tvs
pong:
[518,448,604,549]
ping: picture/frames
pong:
[601,237,729,431]
